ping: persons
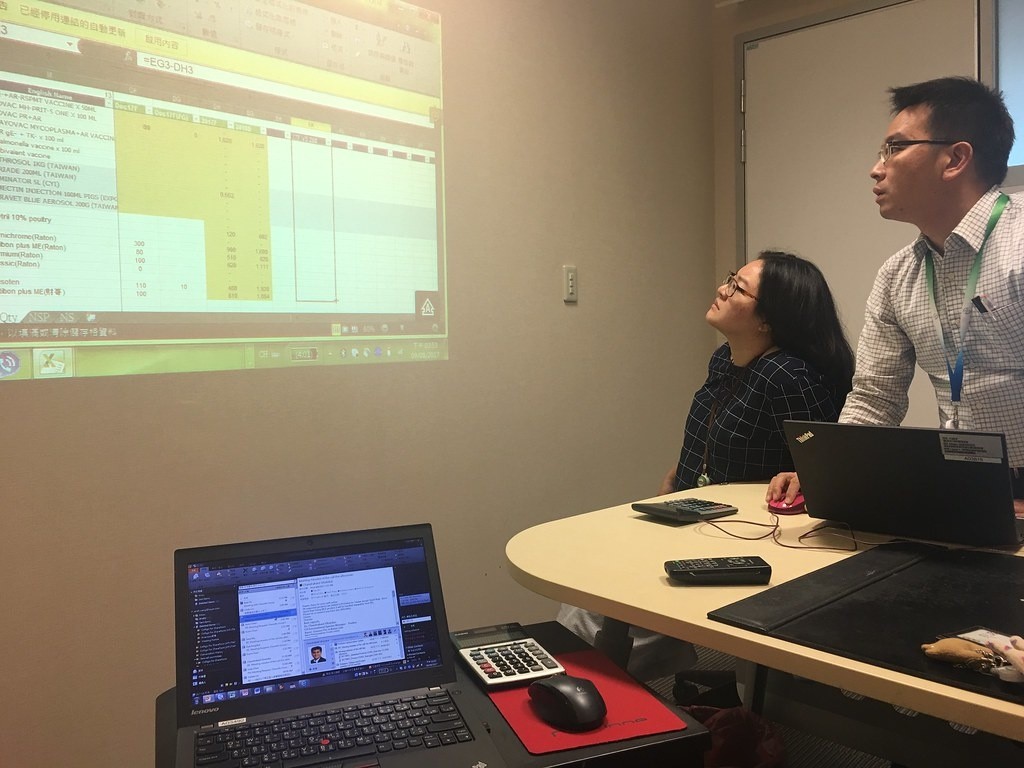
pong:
[656,247,857,506]
[311,646,326,664]
[764,73,1024,516]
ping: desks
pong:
[506,482,1023,768]
[155,619,710,768]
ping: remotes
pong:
[664,555,773,585]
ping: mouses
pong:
[768,492,806,515]
[528,673,608,732]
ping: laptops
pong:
[783,419,1024,548]
[172,524,510,768]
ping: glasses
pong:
[878,139,957,165]
[724,270,756,303]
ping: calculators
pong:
[631,498,739,522]
[450,621,566,689]
